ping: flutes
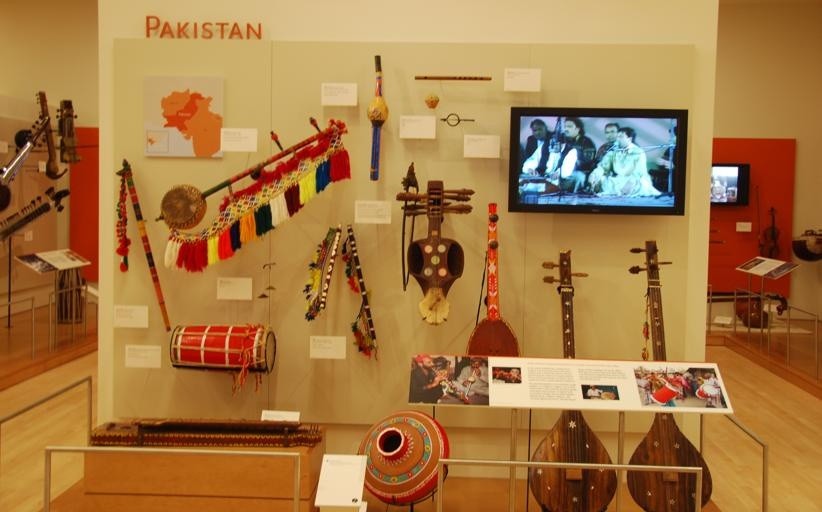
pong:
[414,76,491,80]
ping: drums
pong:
[170,325,276,373]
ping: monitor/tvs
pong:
[710,164,749,204]
[508,106,689,216]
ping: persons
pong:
[410,355,489,406]
[523,116,676,202]
[506,368,520,383]
[639,365,723,409]
[586,385,603,399]
[711,173,727,200]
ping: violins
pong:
[761,208,780,259]
